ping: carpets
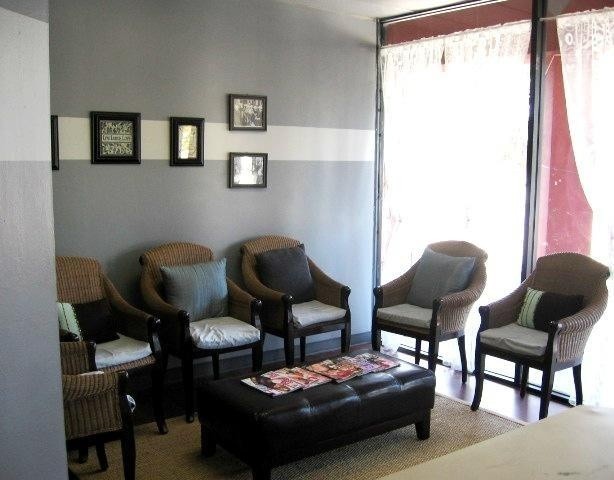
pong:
[66,387,530,480]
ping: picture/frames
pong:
[228,152,267,188]
[89,111,141,164]
[52,115,59,170]
[170,116,204,166]
[229,94,267,130]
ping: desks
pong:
[377,405,614,480]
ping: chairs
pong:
[471,252,610,420]
[241,235,351,371]
[59,342,136,480]
[139,242,265,423]
[371,241,488,382]
[56,257,169,434]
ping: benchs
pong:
[201,349,436,480]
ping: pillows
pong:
[56,298,120,344]
[160,258,230,323]
[405,246,476,308]
[516,287,584,333]
[255,244,317,304]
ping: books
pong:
[240,355,400,399]
